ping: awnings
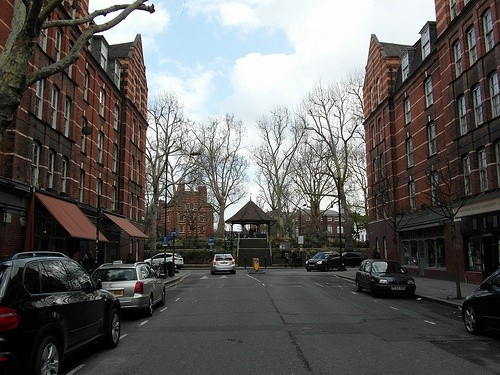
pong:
[103,213,149,239]
[34,192,110,242]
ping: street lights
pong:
[164,151,201,278]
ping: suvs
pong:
[0,249,122,375]
[304,252,341,272]
[91,261,167,317]
[144,252,184,269]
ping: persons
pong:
[74,252,137,278]
[282,251,297,267]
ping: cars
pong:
[354,259,417,299]
[462,270,500,334]
[209,253,236,274]
